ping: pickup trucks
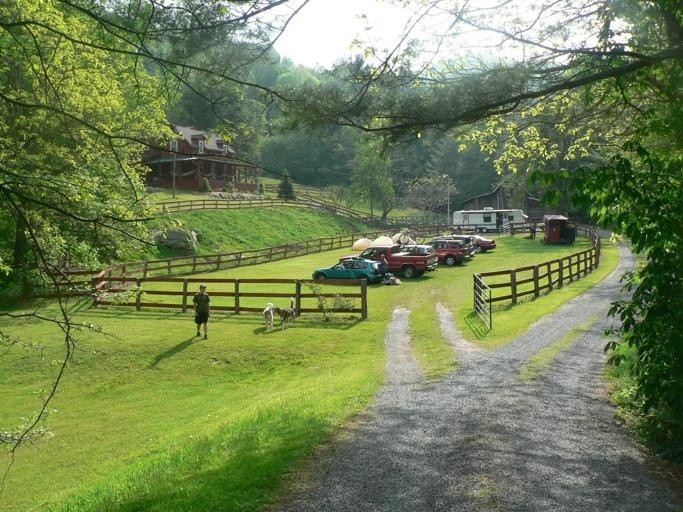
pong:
[339,245,438,279]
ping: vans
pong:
[422,240,469,266]
[431,234,476,261]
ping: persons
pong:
[380,254,387,277]
[456,225,462,232]
[192,284,209,339]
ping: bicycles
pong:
[398,229,425,245]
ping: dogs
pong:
[271,296,295,330]
[262,303,273,328]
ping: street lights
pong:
[442,173,449,230]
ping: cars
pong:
[399,245,433,255]
[311,255,382,286]
[453,234,495,253]
